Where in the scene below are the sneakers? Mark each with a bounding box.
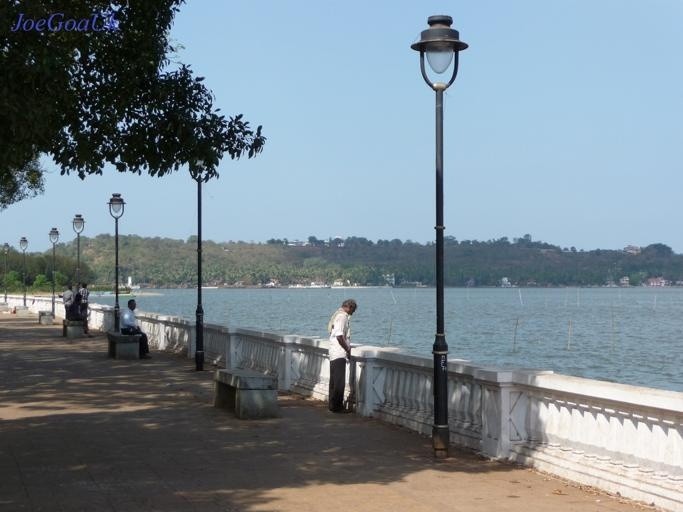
[330,407,351,413]
[140,354,152,359]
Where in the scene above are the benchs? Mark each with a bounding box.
[39,311,52,324]
[215,368,277,418]
[106,331,142,359]
[63,319,85,338]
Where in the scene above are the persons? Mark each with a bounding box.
[327,298,357,414]
[79,284,89,315]
[66,293,88,334]
[62,285,73,312]
[119,299,153,359]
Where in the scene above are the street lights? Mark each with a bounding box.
[106,193,127,333]
[408,13,469,466]
[70,214,87,294]
[3,242,10,303]
[19,236,29,307]
[190,154,214,375]
[47,226,62,318]
